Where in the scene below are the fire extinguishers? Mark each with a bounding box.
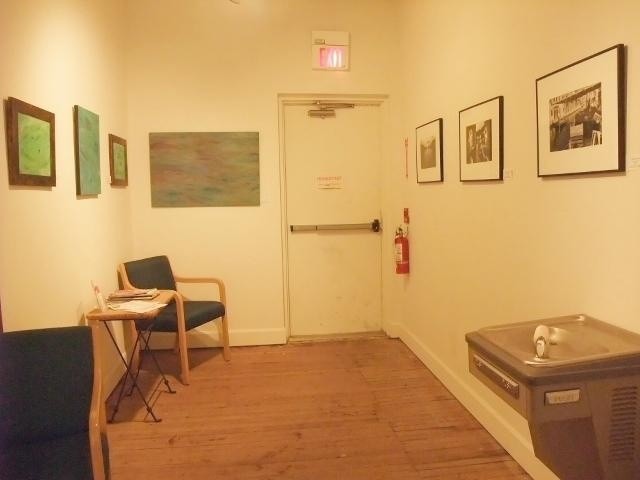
[395,226,409,274]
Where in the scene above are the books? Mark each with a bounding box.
[108,287,168,314]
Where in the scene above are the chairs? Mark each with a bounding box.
[0,326,113,480]
[118,255,230,385]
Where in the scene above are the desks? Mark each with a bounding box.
[87,291,177,422]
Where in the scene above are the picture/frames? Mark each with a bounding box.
[109,134,128,186]
[6,96,56,186]
[459,96,503,182]
[535,43,625,178]
[416,118,442,183]
